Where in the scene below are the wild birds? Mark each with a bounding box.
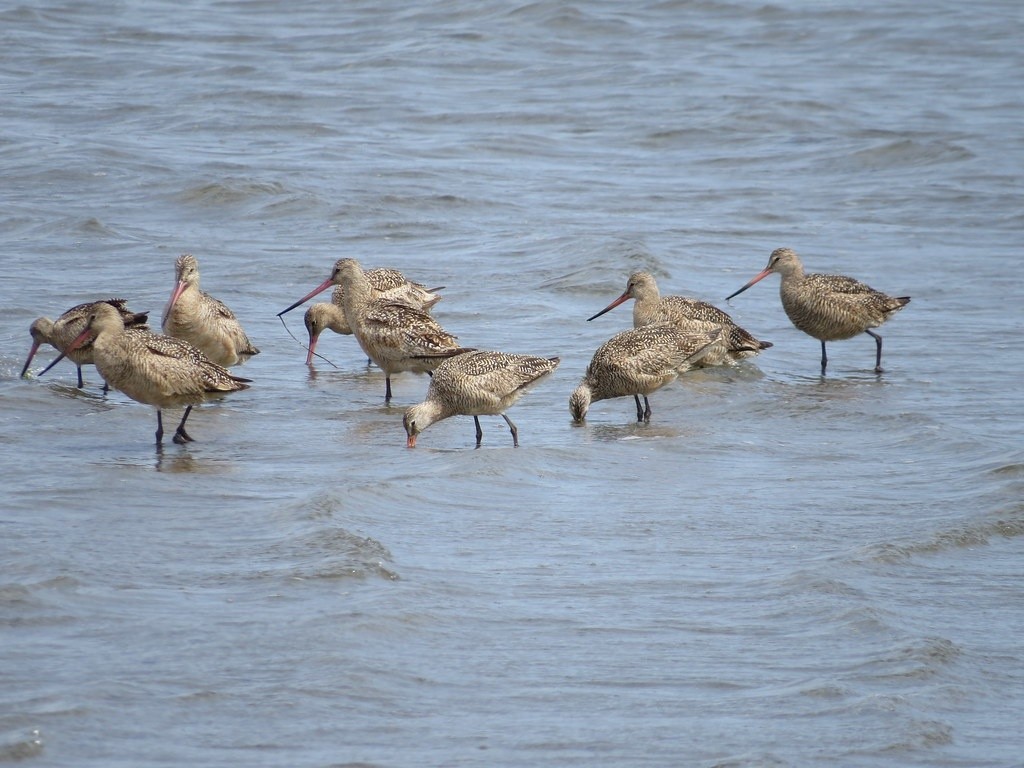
[21,246,910,446]
